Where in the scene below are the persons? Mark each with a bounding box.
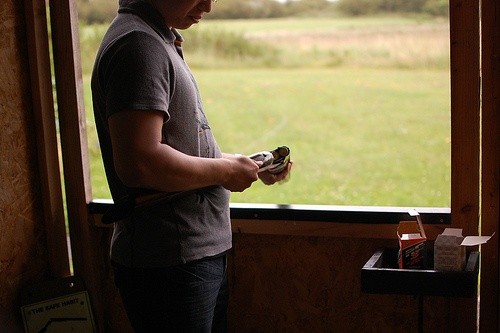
[90,0,292,333]
